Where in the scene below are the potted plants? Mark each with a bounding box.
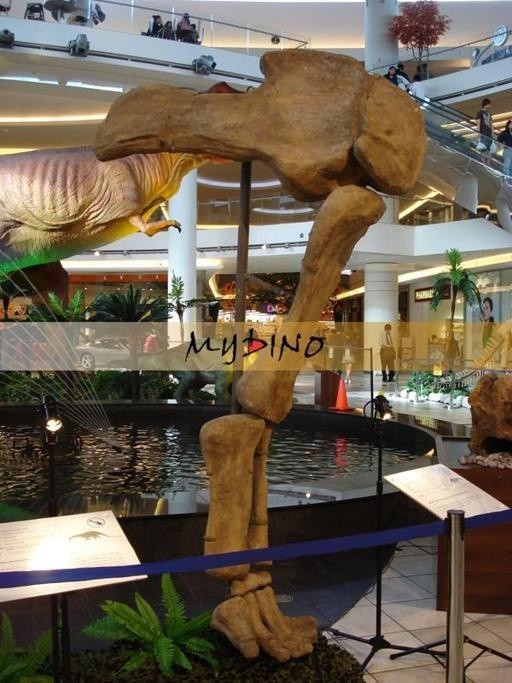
[396,367,473,409]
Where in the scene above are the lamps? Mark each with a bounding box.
[0,27,264,84]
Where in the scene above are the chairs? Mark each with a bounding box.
[138,23,208,45]
[24,2,44,21]
[0,0,12,16]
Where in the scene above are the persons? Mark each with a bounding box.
[143,328,162,353]
[443,332,460,375]
[475,99,494,166]
[476,298,497,349]
[496,120,512,178]
[384,62,432,93]
[315,328,356,386]
[150,12,200,44]
[379,323,398,383]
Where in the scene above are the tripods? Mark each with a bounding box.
[390,635,512,670]
[332,421,447,670]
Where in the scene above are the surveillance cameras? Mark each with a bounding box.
[198,55,216,69]
[75,34,87,54]
[0,29,15,48]
